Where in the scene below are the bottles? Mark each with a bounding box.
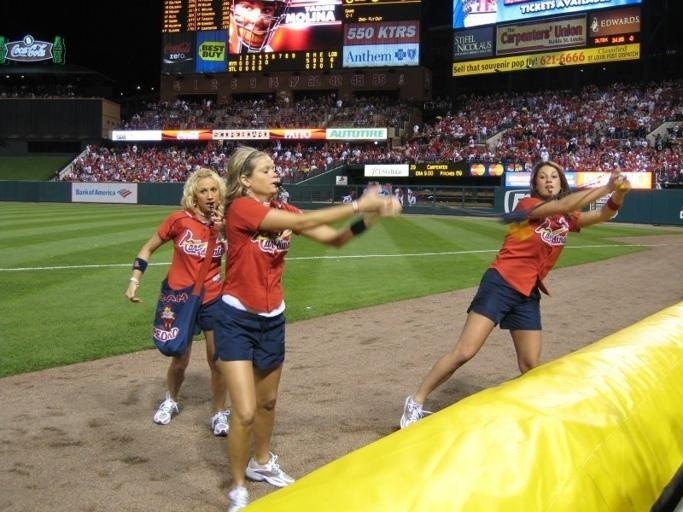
[54,37,63,63]
[0,37,7,66]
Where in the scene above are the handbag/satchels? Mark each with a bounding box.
[152,276,202,358]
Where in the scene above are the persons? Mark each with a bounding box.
[61,83,682,207]
[398,160,633,432]
[212,147,404,512]
[125,166,230,436]
[229,0,290,55]
[2,91,63,99]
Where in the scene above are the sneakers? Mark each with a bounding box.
[212,410,231,436]
[152,390,179,425]
[400,395,434,431]
[245,452,296,488]
[228,488,248,512]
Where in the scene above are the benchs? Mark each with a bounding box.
[63,77,683,213]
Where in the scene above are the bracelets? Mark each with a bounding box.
[606,197,619,211]
[132,258,147,273]
[352,199,360,216]
[129,278,138,285]
[349,217,365,235]
